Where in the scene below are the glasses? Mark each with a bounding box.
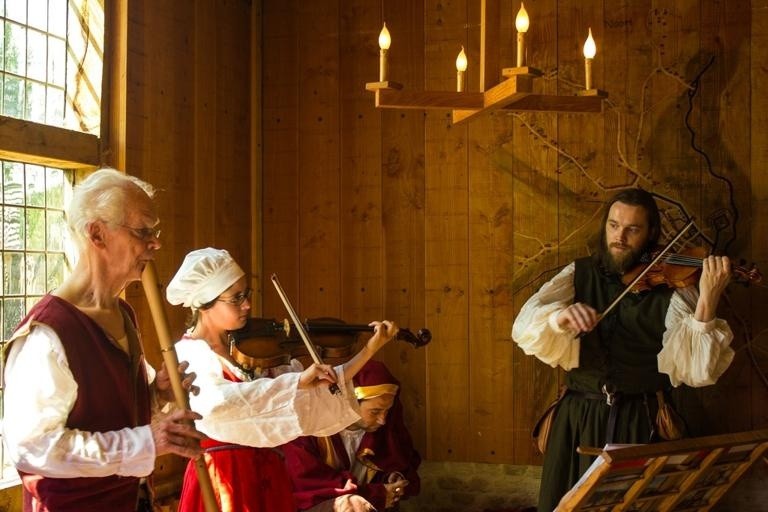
[214,288,253,306]
[101,220,162,242]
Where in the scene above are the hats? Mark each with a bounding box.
[166,247,246,310]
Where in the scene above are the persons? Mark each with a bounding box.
[280,358,423,511]
[509,187,737,512]
[0,166,210,512]
[165,244,401,512]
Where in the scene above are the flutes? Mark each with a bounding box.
[140,260,221,512]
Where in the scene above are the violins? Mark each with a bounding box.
[622,245,764,294]
[226,318,431,371]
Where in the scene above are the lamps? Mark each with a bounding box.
[364,1,609,125]
